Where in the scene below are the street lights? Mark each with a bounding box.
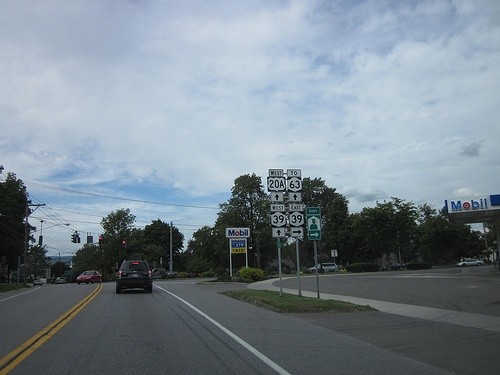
[24,224,70,282]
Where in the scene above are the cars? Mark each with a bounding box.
[382,263,404,271]
[76,271,102,284]
[457,259,484,267]
[32,280,43,286]
[308,262,337,272]
[55,277,66,284]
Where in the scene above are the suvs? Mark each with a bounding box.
[115,259,153,294]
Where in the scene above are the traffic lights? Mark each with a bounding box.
[71,233,81,243]
